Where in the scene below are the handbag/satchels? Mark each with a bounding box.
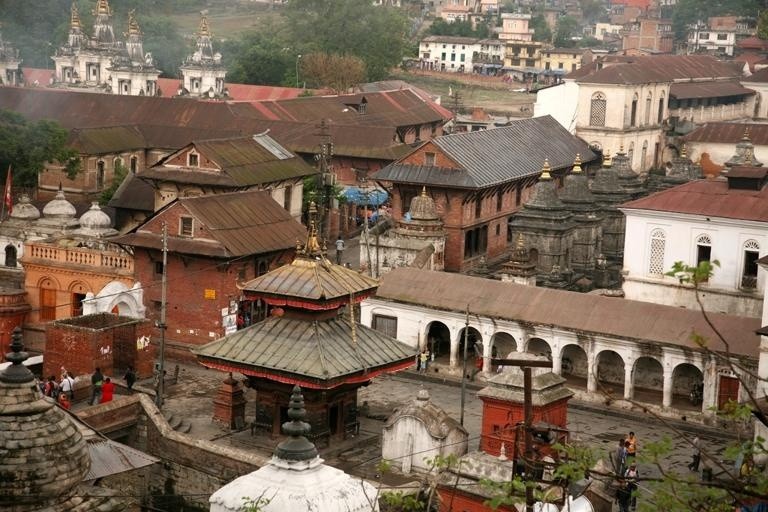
[71,389,74,400]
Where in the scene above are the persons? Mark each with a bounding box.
[334,236,344,265]
[623,461,640,511]
[99,377,116,403]
[420,351,427,370]
[687,432,703,472]
[236,313,245,330]
[153,370,167,407]
[624,431,636,458]
[417,354,421,370]
[123,365,136,395]
[622,441,630,463]
[37,370,75,411]
[616,438,625,471]
[87,367,103,405]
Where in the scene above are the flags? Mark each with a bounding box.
[2,163,13,216]
